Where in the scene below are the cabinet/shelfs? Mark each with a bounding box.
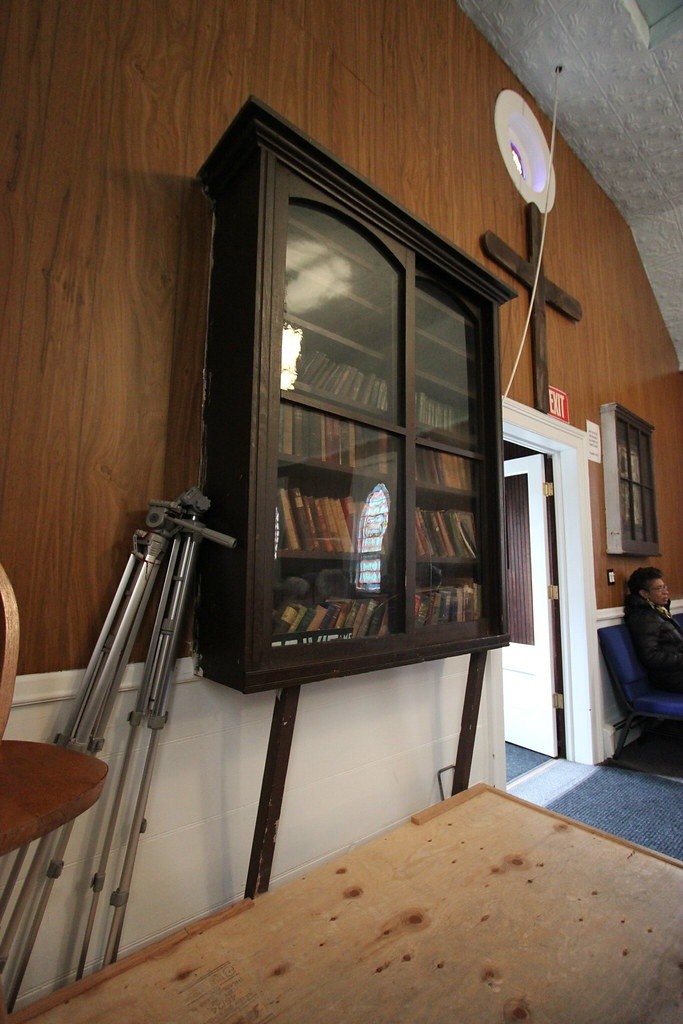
[194,95,519,693]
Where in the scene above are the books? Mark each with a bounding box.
[278,485,478,559]
[279,403,473,491]
[294,345,466,435]
[274,575,482,646]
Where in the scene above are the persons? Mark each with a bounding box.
[622,566,683,696]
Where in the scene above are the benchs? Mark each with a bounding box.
[596,612,683,760]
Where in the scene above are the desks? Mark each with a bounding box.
[1,783,683,1024]
[0,738,112,856]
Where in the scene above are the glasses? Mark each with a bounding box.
[649,585,668,591]
